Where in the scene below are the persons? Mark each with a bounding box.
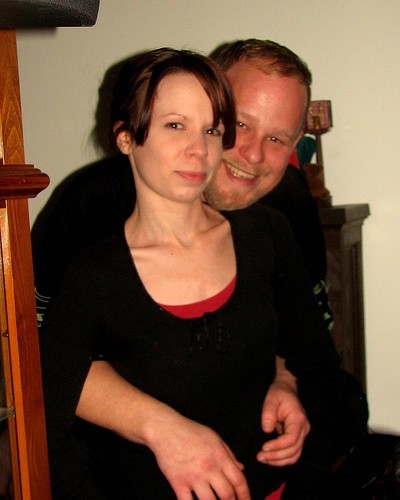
[44,45,329,499]
[1,36,399,500]
